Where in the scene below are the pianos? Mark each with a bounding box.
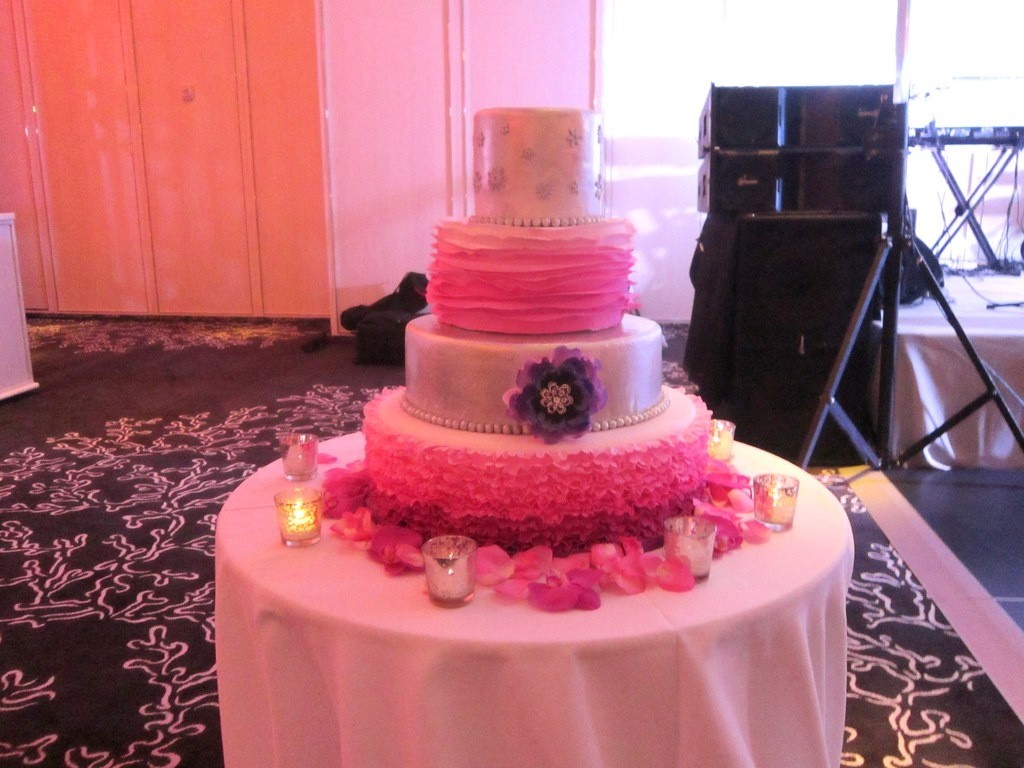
[908,126,1024,149]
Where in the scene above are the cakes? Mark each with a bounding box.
[362,110,714,558]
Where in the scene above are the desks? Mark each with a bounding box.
[214,430,857,768]
